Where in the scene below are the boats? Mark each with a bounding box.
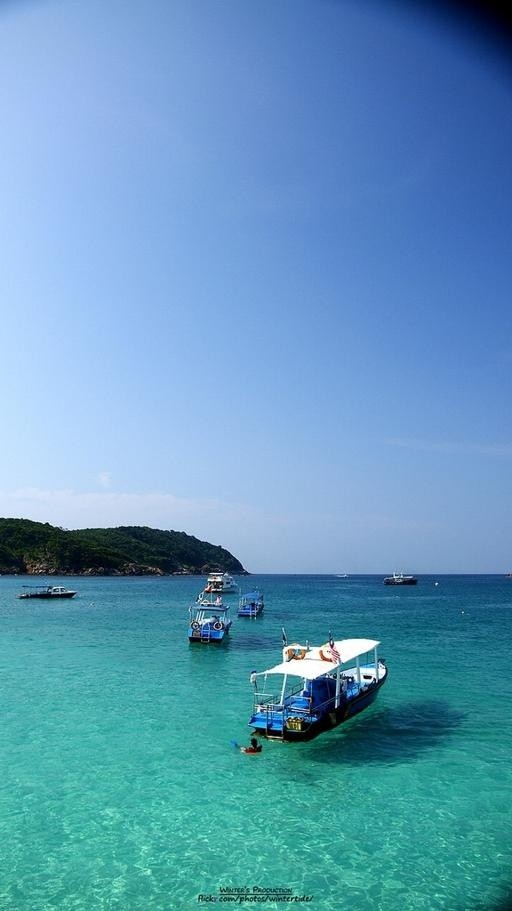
[236,592,264,617]
[383,573,418,586]
[16,586,78,600]
[248,628,388,742]
[207,573,237,592]
[187,586,233,644]
[337,573,349,578]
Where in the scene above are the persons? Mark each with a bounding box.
[332,672,344,686]
[242,738,262,754]
[216,595,222,602]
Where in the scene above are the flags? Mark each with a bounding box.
[329,630,340,661]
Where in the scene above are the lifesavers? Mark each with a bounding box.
[287,644,305,660]
[213,621,223,630]
[191,621,199,630]
[320,644,332,662]
